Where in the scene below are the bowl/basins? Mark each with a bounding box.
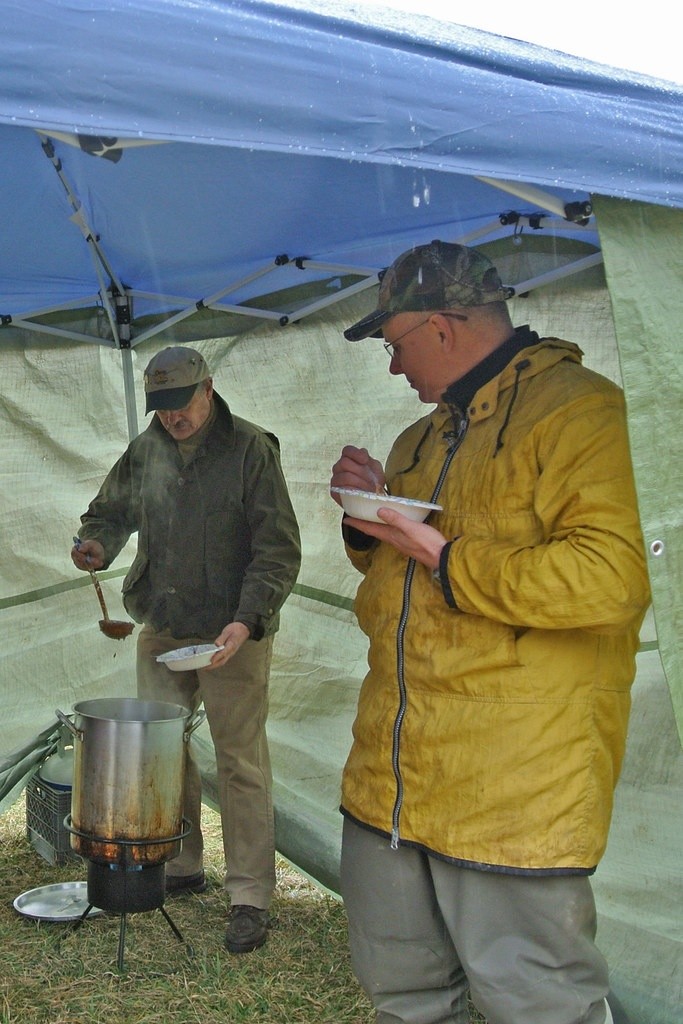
[156,643,225,671]
[331,487,443,524]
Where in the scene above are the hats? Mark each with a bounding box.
[143,344,210,416]
[343,241,516,342]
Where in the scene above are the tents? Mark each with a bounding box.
[0,0,683,1024]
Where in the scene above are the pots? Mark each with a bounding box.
[44,699,206,864]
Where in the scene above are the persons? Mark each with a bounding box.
[71,348,301,953]
[330,243,652,1024]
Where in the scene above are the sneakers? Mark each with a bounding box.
[225,903,269,953]
[162,870,208,895]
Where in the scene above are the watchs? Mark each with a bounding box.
[432,569,442,587]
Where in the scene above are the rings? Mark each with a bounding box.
[74,560,76,564]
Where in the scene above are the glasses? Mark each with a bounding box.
[383,313,468,358]
